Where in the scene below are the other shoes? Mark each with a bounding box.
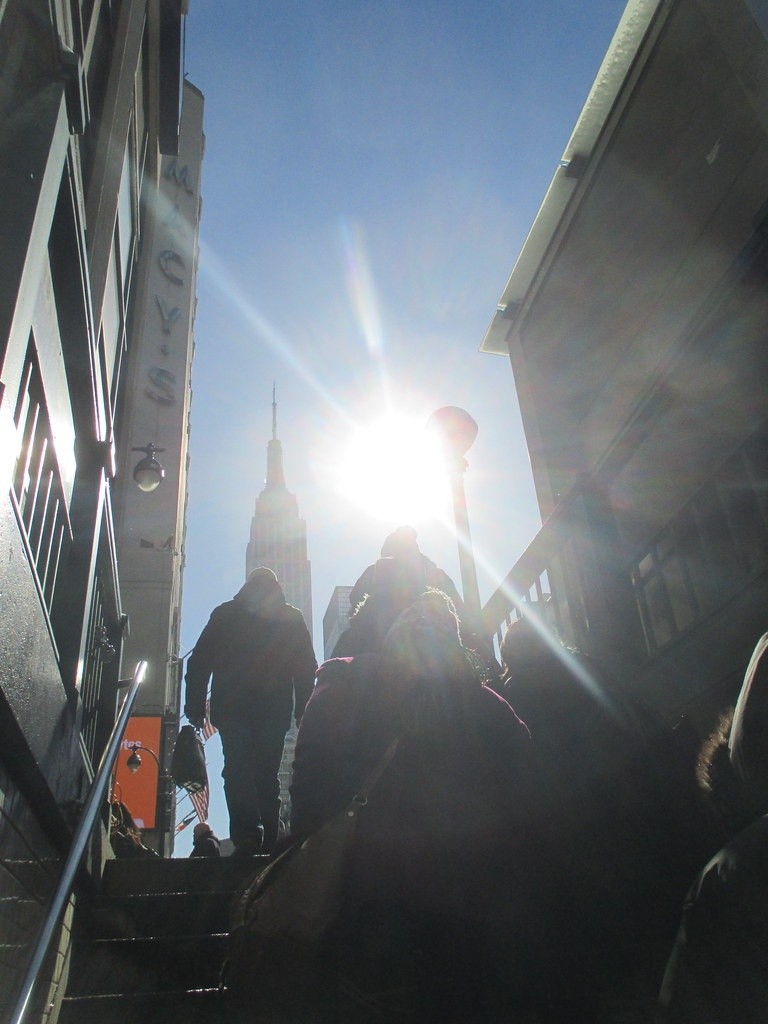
[229,831,263,857]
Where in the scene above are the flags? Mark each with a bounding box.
[176,816,196,831]
[202,697,219,742]
[189,780,209,823]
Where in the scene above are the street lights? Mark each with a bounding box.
[125,743,169,859]
[430,407,481,615]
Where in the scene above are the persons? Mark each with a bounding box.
[289,523,768,1024]
[184,567,318,855]
[189,823,220,858]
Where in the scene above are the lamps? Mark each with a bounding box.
[130,444,167,493]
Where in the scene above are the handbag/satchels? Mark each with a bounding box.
[224,792,369,942]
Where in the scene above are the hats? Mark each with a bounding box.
[193,823,212,837]
[380,525,420,557]
[499,617,545,661]
[247,566,277,582]
[383,585,462,648]
